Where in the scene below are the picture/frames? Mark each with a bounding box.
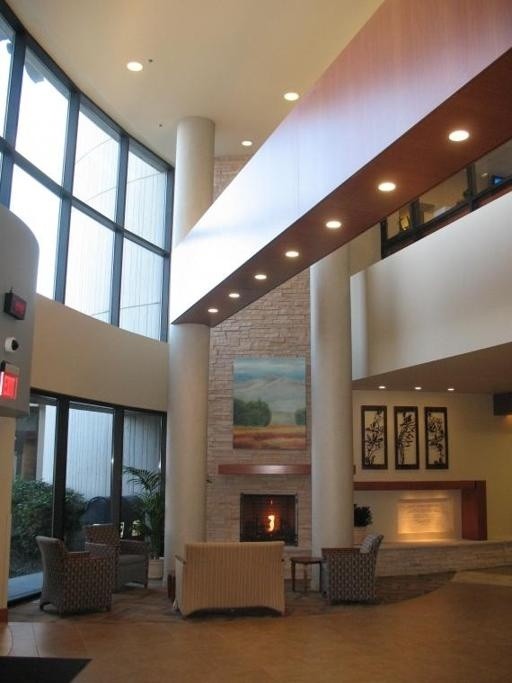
[360,404,448,470]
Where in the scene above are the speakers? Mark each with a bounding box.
[493,390,512,415]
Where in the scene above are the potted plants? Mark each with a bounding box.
[122,464,165,580]
[353,503,373,545]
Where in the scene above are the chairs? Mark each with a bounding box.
[321,533,384,606]
[35,522,150,618]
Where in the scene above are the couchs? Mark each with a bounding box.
[172,541,286,619]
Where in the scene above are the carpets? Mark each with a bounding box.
[0,655,92,682]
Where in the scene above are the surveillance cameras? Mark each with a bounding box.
[5,337,20,352]
